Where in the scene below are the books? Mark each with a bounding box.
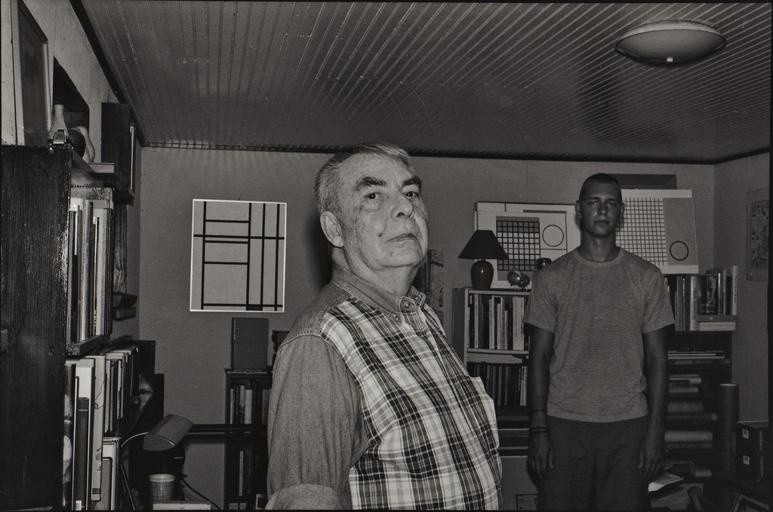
[660,265,740,479]
[230,384,272,511]
[465,293,528,407]
[230,318,268,371]
[63,197,138,510]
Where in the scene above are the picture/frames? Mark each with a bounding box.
[188,197,288,314]
[9,0,53,147]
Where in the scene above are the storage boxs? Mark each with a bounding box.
[735,418,771,486]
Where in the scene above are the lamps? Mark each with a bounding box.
[119,413,196,511]
[611,19,727,67]
[453,230,511,291]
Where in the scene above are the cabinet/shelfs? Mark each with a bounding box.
[663,321,735,446]
[2,143,167,509]
[453,285,554,426]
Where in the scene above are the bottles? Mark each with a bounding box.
[47,104,68,144]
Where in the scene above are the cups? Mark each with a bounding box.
[147,472,174,506]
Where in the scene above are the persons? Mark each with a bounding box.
[267,143,503,509]
[523,174,675,511]
